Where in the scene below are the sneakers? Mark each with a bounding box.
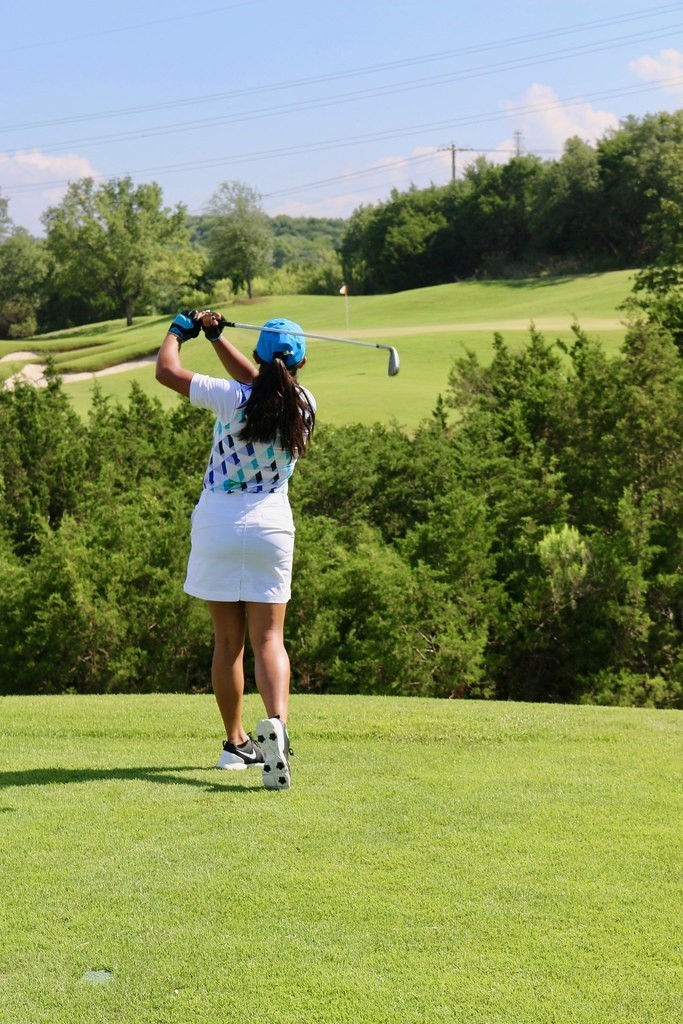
[216,731,265,770]
[257,714,294,790]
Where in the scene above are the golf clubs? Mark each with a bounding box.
[220,318,401,379]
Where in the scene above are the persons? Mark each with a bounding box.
[155,310,317,795]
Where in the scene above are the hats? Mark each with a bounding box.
[256,318,307,367]
